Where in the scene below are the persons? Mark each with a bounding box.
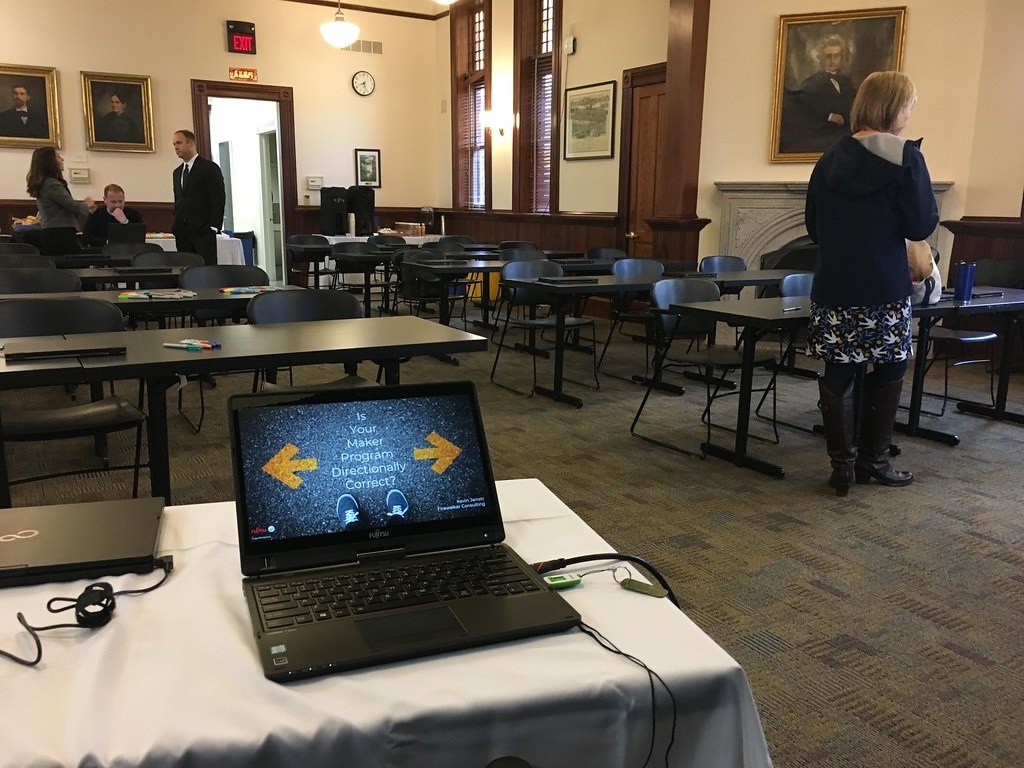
[26,146,98,232]
[82,184,143,247]
[805,71,940,497]
[172,130,226,266]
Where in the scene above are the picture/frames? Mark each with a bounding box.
[355,148,381,188]
[0,63,62,150]
[561,80,617,160]
[769,6,909,163]
[80,70,155,155]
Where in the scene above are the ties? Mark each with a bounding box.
[183,164,189,188]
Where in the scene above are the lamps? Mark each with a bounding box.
[320,0,360,51]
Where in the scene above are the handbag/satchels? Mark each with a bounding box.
[905,238,941,307]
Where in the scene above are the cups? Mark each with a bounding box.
[954,260,977,302]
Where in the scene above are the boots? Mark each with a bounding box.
[818,377,857,497]
[855,371,914,485]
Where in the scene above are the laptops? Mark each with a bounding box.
[367,243,468,266]
[548,259,594,264]
[114,266,172,273]
[4,336,126,360]
[0,497,167,588]
[942,288,1004,298]
[662,271,717,278]
[108,223,147,245]
[228,381,582,683]
[539,277,599,284]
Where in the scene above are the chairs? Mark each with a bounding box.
[755,273,836,431]
[0,225,749,453]
[894,324,997,417]
[630,277,780,460]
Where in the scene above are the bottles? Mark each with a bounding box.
[395,222,425,236]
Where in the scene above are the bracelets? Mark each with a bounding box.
[124,219,129,224]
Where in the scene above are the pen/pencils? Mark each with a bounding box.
[163,339,222,352]
[783,306,802,312]
[117,289,197,299]
[219,286,284,293]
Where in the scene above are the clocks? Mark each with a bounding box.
[351,71,376,96]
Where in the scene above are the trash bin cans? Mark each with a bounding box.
[222,229,255,266]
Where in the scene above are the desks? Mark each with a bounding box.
[399,261,617,366]
[331,251,583,315]
[0,313,488,506]
[1,478,773,768]
[503,269,804,407]
[319,234,447,264]
[0,284,312,333]
[146,236,246,265]
[63,266,191,288]
[288,244,501,291]
[668,288,1024,478]
